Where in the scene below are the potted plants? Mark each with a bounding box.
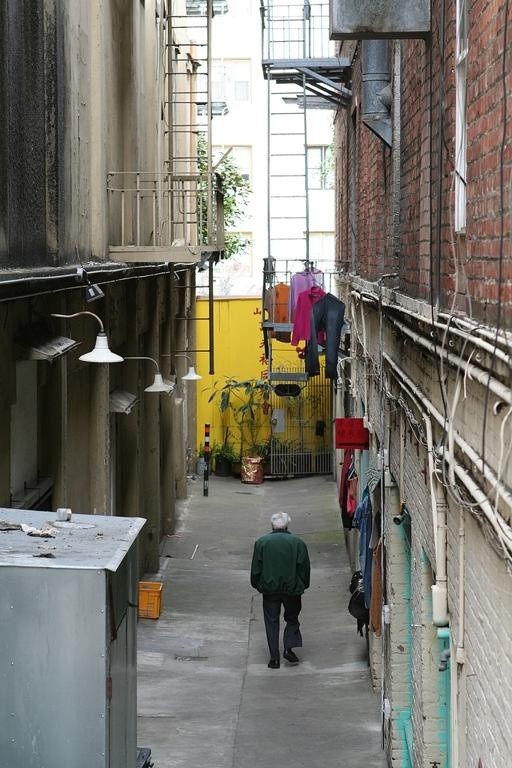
[196,376,331,483]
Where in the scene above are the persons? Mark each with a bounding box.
[249,512,312,669]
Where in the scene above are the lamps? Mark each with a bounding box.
[393,502,408,525]
[123,355,175,393]
[51,311,122,365]
[174,351,202,381]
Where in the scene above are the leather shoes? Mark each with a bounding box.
[267,660,281,668]
[284,649,299,664]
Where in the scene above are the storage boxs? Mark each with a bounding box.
[138,581,163,620]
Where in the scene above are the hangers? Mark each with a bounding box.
[348,455,357,469]
[307,278,324,298]
[302,261,313,273]
[349,467,360,479]
[276,275,287,284]
